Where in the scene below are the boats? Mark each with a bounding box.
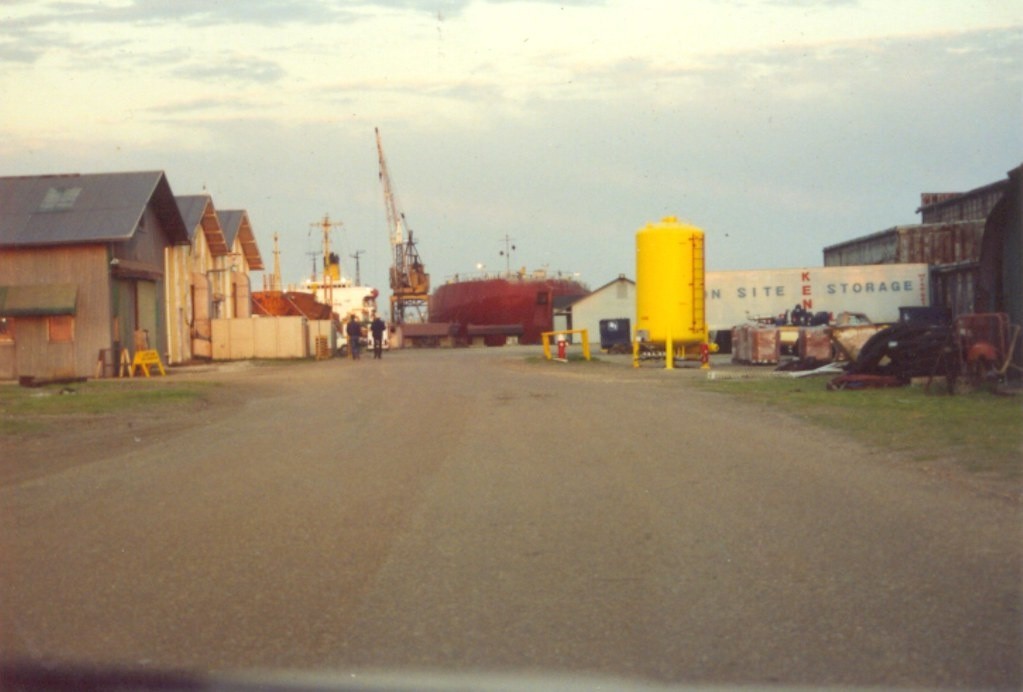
[429,232,595,347]
[283,212,379,323]
[251,232,343,333]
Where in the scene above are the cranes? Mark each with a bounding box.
[373,126,430,295]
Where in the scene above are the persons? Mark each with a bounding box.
[346,313,361,360]
[371,313,386,358]
